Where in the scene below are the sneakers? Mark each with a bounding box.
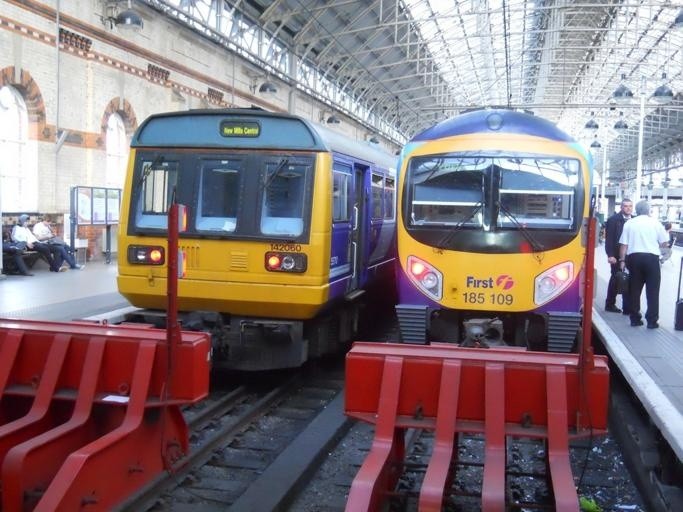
[59,266,66,272]
[623,310,630,315]
[631,321,643,326]
[648,323,659,328]
[23,271,33,275]
[605,304,621,312]
[10,245,22,255]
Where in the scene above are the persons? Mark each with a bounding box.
[656,223,672,266]
[619,199,670,328]
[605,199,636,315]
[11,214,69,272]
[3,231,35,276]
[33,214,85,272]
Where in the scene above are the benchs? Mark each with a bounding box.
[2,223,77,272]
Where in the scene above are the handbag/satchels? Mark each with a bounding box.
[614,272,629,294]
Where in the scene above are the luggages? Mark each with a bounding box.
[674,256,683,330]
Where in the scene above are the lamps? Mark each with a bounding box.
[101,8,142,30]
[584,70,673,147]
[364,132,379,144]
[253,78,277,94]
[322,111,341,123]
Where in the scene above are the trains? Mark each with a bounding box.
[394,108,599,354]
[118,104,397,372]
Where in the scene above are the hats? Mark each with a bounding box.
[19,214,28,223]
